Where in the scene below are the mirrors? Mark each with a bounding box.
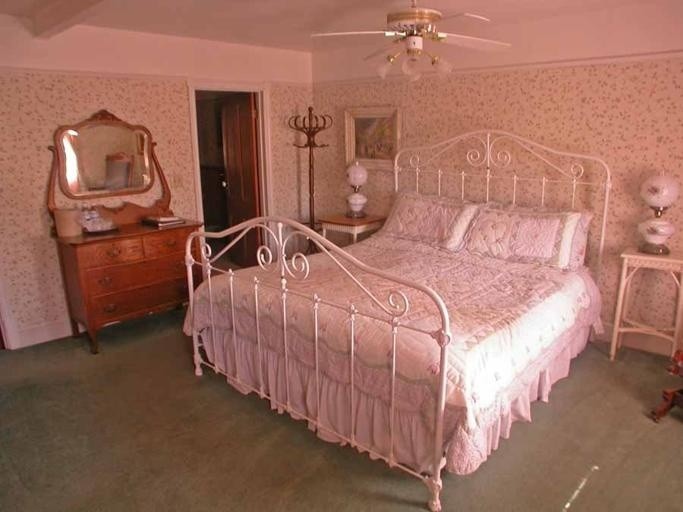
[53,109,156,199]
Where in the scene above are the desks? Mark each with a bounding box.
[607,248,683,360]
[318,214,383,243]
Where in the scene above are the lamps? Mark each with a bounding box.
[373,35,450,82]
[346,163,368,218]
[640,171,680,259]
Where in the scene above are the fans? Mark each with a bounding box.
[309,0,511,82]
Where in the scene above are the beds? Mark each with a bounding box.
[180,129,612,512]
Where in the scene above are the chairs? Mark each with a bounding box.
[103,149,133,192]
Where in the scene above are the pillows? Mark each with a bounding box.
[388,192,479,251]
[461,202,589,269]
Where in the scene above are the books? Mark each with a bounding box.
[144,216,181,221]
[141,220,184,227]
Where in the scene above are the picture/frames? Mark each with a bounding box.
[345,107,401,172]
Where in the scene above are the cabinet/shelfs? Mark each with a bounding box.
[55,218,203,355]
